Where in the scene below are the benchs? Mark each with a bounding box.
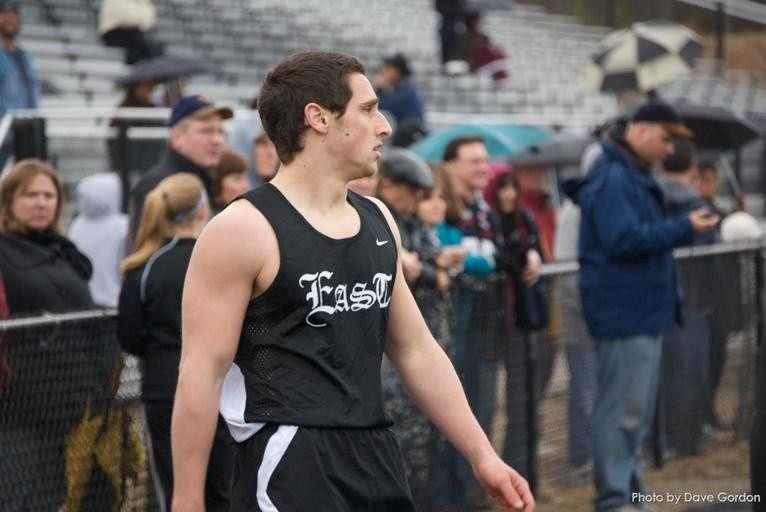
[1,1,765,234]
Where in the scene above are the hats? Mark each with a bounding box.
[378,147,436,192]
[629,102,695,138]
[381,54,412,77]
[166,93,234,128]
